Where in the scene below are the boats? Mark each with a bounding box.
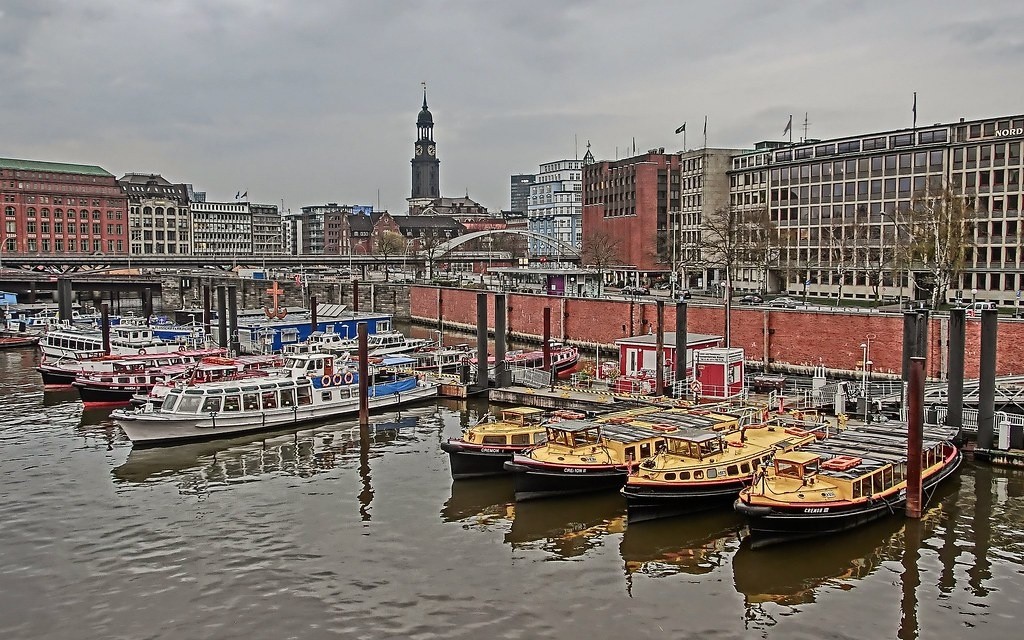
[441,401,628,479]
[733,422,965,537]
[0,296,582,454]
[504,405,742,501]
[620,409,827,526]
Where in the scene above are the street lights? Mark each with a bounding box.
[487,226,493,268]
[347,237,352,281]
[668,210,682,302]
[403,237,420,283]
[880,211,902,313]
[553,219,564,268]
[262,234,281,273]
[570,277,574,296]
[971,288,978,316]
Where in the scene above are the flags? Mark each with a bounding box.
[912,94,916,122]
[241,190,247,198]
[676,124,685,134]
[235,192,239,199]
[783,120,790,136]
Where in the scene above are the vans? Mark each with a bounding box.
[965,303,997,317]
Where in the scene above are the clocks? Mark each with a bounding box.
[415,145,423,156]
[427,145,436,157]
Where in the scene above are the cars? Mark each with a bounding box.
[739,295,764,306]
[653,283,661,290]
[661,283,679,290]
[670,290,691,300]
[769,297,797,307]
[633,288,650,295]
[620,286,636,294]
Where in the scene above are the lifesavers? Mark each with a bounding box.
[178,345,186,352]
[321,375,333,387]
[690,380,702,393]
[138,349,147,355]
[187,345,194,350]
[344,372,354,384]
[333,374,344,386]
